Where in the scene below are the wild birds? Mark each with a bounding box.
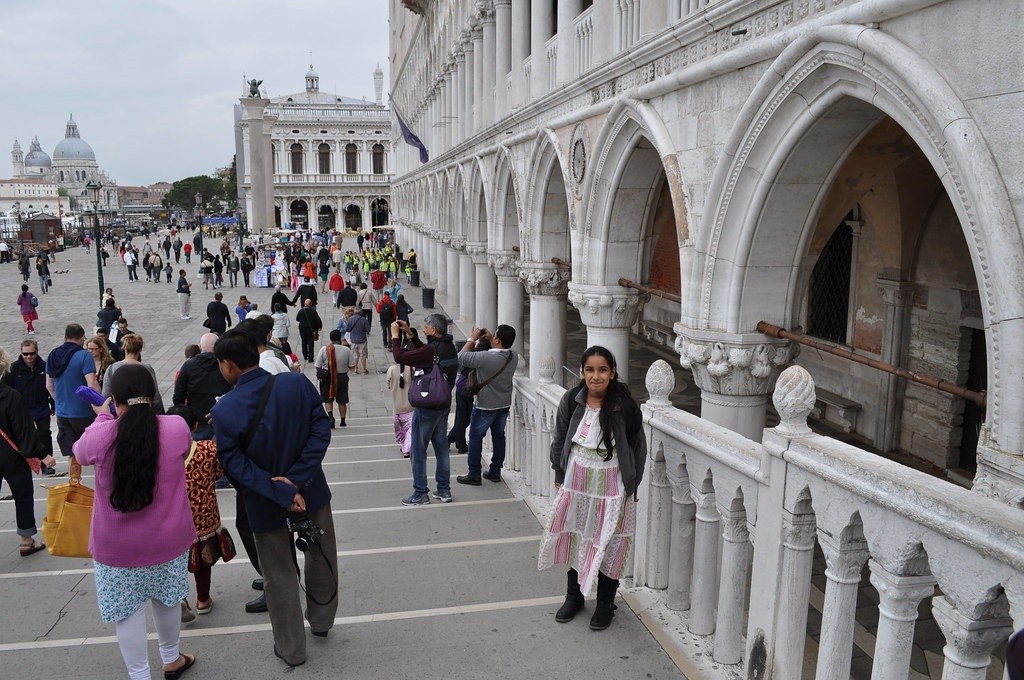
[54,269,71,275]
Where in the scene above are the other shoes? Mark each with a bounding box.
[330,417,335,428]
[181,314,192,319]
[458,445,468,454]
[42,466,55,475]
[333,303,336,307]
[129,276,160,283]
[26,331,35,334]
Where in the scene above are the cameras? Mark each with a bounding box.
[286,509,324,551]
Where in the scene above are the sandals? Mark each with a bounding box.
[19,539,45,556]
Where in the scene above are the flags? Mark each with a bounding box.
[392,104,429,164]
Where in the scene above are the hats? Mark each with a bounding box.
[240,295,246,300]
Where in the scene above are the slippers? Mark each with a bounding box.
[164,578,328,680]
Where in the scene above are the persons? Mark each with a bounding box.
[84,235,92,252]
[0,314,356,680]
[538,345,647,630]
[17,284,39,334]
[95,220,417,374]
[386,314,518,506]
[0,232,64,293]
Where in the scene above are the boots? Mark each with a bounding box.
[556,569,584,623]
[590,570,620,630]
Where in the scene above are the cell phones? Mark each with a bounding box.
[478,329,486,336]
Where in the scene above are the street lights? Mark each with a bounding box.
[14,201,24,252]
[86,179,104,309]
[236,206,244,252]
[194,191,203,263]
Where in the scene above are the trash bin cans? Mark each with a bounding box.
[410,271,420,286]
[422,288,435,308]
[401,260,408,272]
[398,253,403,263]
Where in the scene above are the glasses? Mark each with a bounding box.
[22,351,36,356]
[83,336,86,340]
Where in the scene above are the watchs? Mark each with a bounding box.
[466,338,476,344]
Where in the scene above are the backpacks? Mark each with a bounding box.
[380,299,393,322]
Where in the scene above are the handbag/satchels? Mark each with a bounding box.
[40,456,94,558]
[132,259,154,269]
[25,457,42,475]
[408,343,477,409]
[313,331,319,341]
[48,278,52,286]
[203,318,211,329]
[316,368,330,383]
[31,296,38,307]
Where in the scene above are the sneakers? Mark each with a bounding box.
[401,490,452,506]
[457,469,501,485]
